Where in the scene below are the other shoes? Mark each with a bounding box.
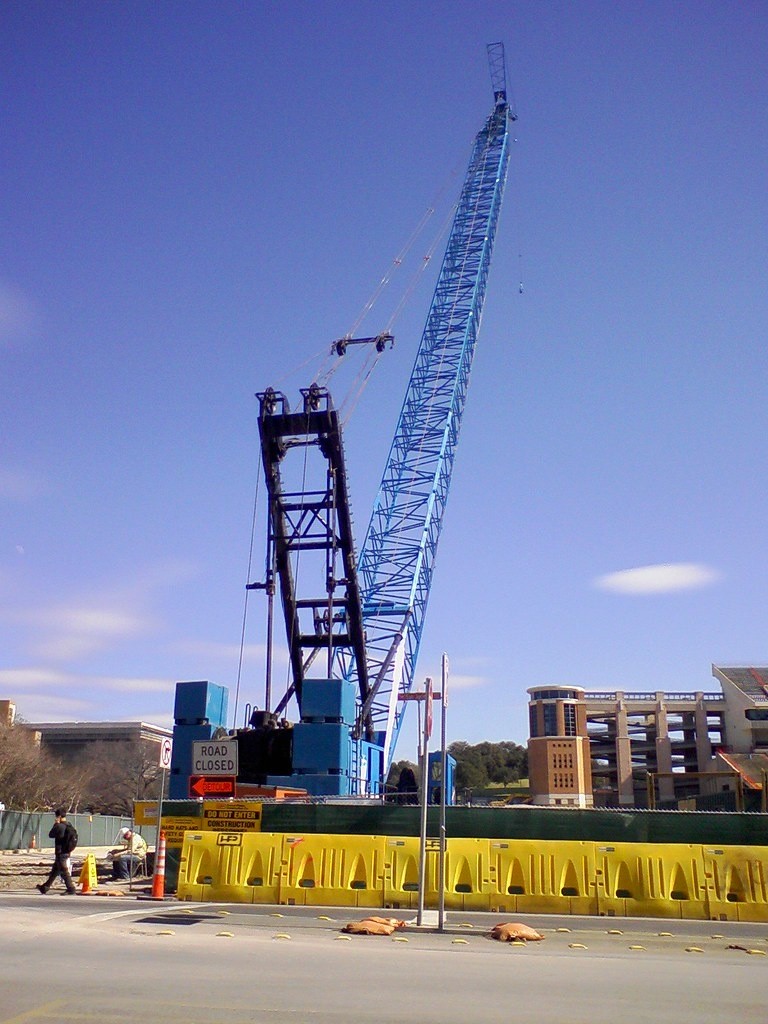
[116,876,130,882]
[60,888,78,896]
[36,883,48,895]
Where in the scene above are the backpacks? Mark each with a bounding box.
[58,821,78,854]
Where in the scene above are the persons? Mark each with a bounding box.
[106,826,147,882]
[36,807,78,896]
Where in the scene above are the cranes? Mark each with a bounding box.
[186,38,518,800]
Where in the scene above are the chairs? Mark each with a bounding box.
[130,857,147,876]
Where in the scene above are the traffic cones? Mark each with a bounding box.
[29,833,36,849]
[137,829,177,900]
[76,855,101,895]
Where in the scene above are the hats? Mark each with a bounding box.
[119,827,130,838]
